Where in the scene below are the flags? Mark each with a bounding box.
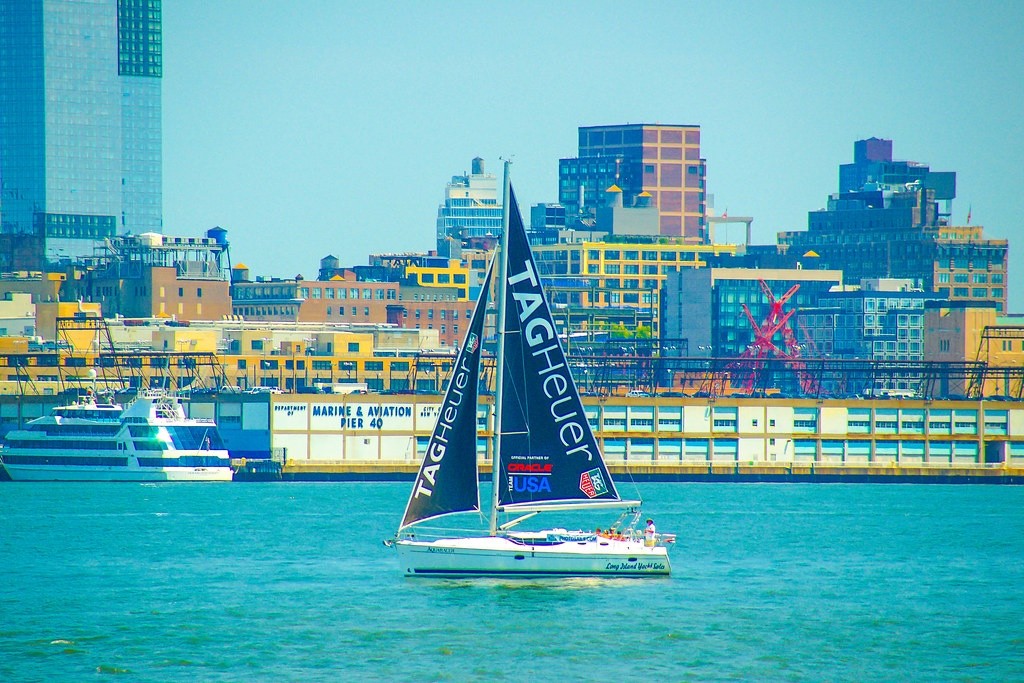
[722,210,727,218]
[967,205,972,224]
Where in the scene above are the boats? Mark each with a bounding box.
[0,388,234,482]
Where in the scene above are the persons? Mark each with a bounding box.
[596,528,625,541]
[644,518,655,547]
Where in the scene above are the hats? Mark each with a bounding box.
[646,518,653,523]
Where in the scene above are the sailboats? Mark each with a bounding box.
[383,157,674,580]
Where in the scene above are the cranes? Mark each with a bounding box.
[735,276,827,394]
